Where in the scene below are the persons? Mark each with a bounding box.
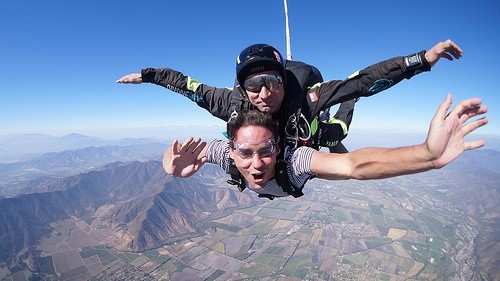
[163,93,490,201]
[114,38,463,152]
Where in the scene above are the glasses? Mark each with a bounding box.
[243,73,283,93]
[232,139,277,159]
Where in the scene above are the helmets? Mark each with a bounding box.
[236,42,286,85]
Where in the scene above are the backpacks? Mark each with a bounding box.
[227,61,322,138]
